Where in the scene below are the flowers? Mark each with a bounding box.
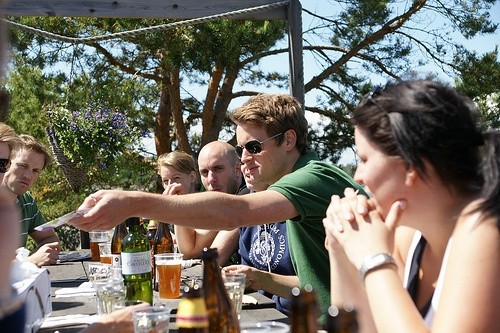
[44,101,151,171]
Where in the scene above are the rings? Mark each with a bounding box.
[90,194,97,202]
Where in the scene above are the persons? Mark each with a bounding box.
[67,93,371,333]
[157,151,205,195]
[209,162,297,315]
[162,142,250,268]
[323,78,500,333]
[0,123,62,296]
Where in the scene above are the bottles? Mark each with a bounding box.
[112,222,129,269]
[175,285,209,333]
[151,221,173,292]
[200,248,241,333]
[146,219,159,288]
[121,217,153,308]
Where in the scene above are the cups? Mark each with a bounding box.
[238,321,291,333]
[109,265,122,281]
[131,305,172,333]
[98,242,112,265]
[221,272,246,318]
[88,230,110,263]
[89,264,112,288]
[93,279,126,316]
[153,253,184,300]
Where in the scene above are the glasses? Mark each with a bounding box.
[0,158,11,172]
[235,131,285,159]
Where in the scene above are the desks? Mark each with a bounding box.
[36,248,289,333]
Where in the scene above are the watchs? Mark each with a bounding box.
[360,254,398,281]
